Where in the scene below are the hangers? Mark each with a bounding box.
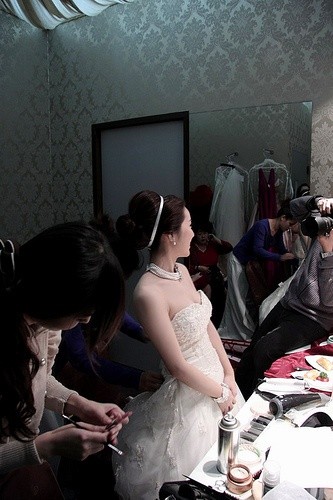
[216,154,249,175]
[249,150,286,172]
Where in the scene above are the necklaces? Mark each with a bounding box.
[146,263,181,280]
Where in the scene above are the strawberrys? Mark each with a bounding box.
[317,372,329,382]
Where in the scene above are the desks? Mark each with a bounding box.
[186,341,333,500]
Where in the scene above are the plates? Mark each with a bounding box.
[291,370,333,392]
[305,355,333,372]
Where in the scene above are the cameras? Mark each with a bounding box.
[300,204,333,237]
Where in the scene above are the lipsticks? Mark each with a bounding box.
[104,413,128,431]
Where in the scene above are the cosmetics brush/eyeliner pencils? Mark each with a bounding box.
[61,414,126,457]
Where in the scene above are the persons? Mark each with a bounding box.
[217,205,302,342]
[234,194,321,402]
[183,222,233,329]
[289,183,312,275]
[0,219,155,500]
[111,189,246,500]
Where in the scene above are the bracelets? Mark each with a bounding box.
[213,383,231,404]
[196,265,199,272]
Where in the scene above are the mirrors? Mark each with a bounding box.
[188,100,313,248]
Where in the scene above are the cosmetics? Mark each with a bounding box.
[224,463,254,495]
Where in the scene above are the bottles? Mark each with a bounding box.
[262,466,281,498]
[217,412,240,474]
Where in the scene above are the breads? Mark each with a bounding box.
[304,370,319,379]
[316,357,333,371]
